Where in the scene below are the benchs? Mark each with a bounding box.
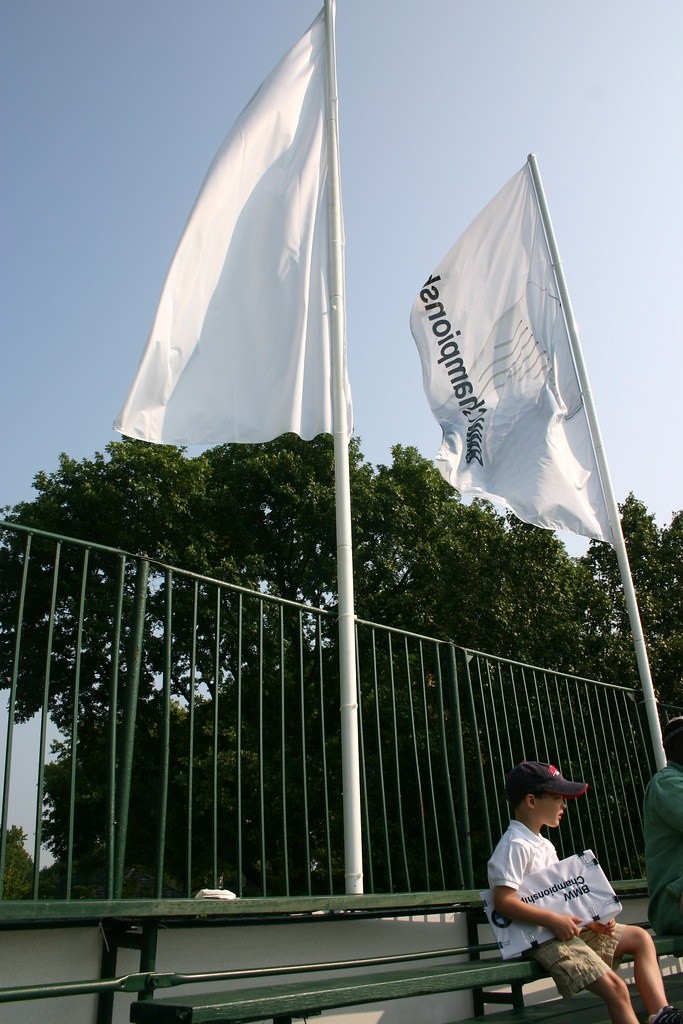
[0,878,683,1024]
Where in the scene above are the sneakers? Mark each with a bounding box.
[648,1005,683,1024]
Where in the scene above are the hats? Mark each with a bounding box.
[507,760,589,803]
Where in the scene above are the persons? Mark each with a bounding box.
[488,762,683,1024]
[643,716,683,934]
